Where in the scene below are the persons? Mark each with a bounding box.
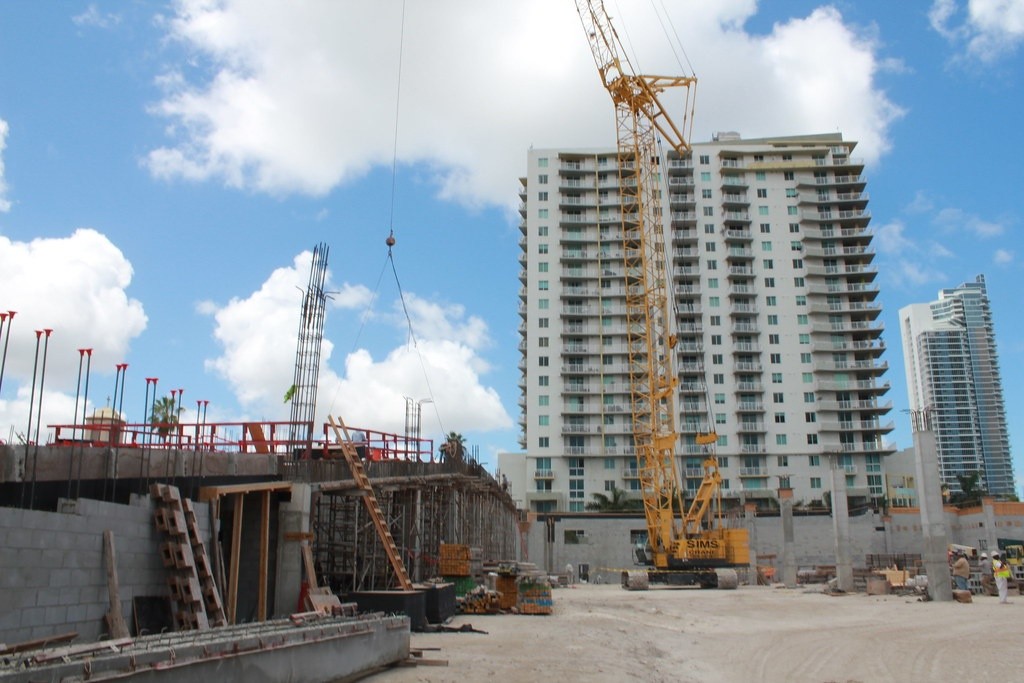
[949,552,971,590]
[978,553,994,595]
[989,550,1011,604]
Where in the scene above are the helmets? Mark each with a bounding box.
[980,553,989,558]
[991,551,1000,557]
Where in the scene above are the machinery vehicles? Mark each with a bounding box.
[571,0,750,590]
